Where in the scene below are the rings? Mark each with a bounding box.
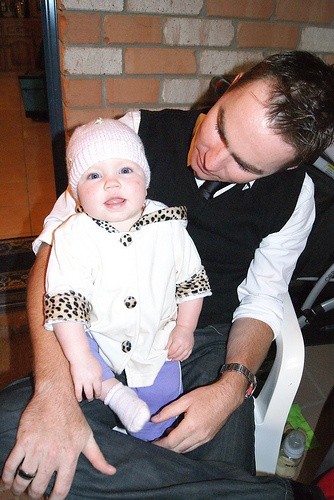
[16,466,36,481]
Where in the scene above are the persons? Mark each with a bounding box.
[0,50,334,500]
[42,116,212,443]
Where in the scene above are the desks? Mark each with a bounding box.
[18,72,49,121]
[0,17,43,69]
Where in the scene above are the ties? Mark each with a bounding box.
[198,180,233,201]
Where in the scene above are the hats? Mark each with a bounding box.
[65,109,150,205]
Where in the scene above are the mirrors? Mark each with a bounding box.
[0,1,71,263]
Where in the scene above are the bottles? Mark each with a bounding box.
[275,430,306,479]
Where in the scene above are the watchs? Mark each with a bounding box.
[218,363,258,401]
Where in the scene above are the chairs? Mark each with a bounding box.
[253,294,306,477]
